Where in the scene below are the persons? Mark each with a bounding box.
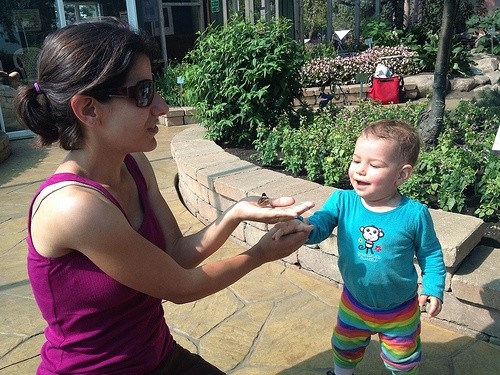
[14,16,319,375]
[271,118,448,375]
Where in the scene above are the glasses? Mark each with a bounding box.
[105,71,157,108]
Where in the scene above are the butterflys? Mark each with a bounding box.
[258,192,269,206]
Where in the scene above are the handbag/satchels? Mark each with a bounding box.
[368,76,405,105]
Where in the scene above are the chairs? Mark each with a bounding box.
[13,46,43,85]
